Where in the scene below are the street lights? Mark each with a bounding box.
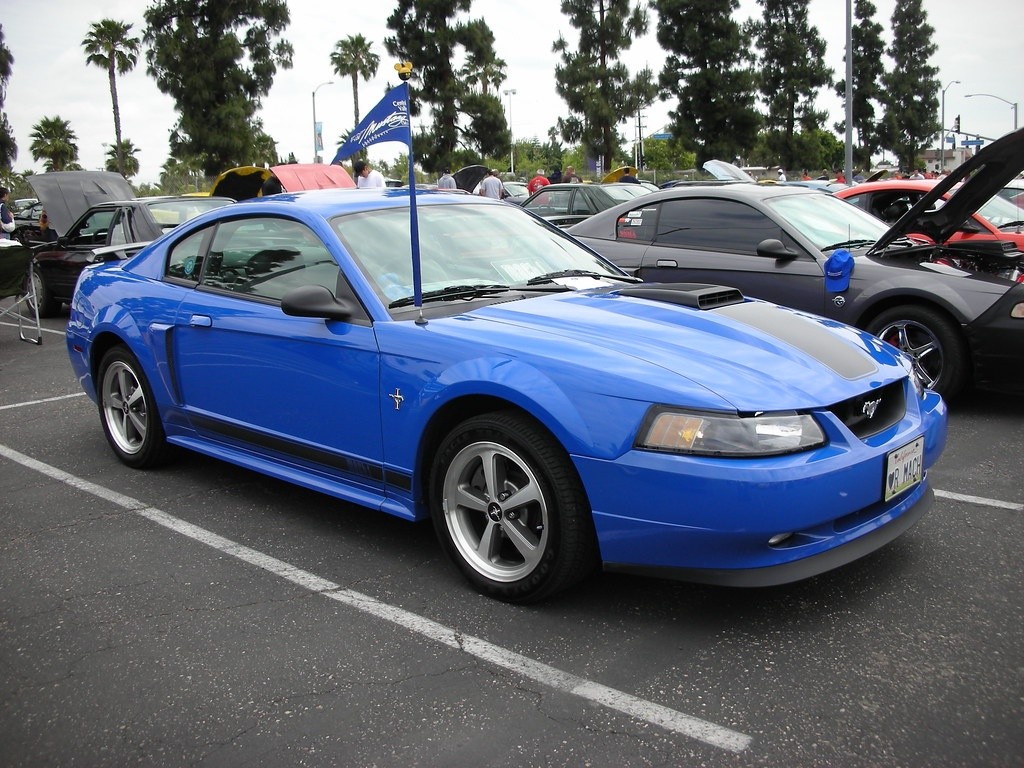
[310,79,335,160]
[964,92,1020,129]
[940,79,961,174]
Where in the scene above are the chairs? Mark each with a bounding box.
[886,201,909,226]
[0,246,42,345]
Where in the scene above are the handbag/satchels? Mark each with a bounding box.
[0,203,15,233]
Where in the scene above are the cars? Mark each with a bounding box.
[834,180,1024,253]
[568,128,1024,407]
[20,172,239,318]
[64,186,949,605]
[521,181,655,229]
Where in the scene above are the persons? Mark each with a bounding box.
[262,161,298,195]
[835,168,845,183]
[438,166,458,189]
[0,187,15,239]
[479,168,506,200]
[562,166,584,183]
[909,163,952,181]
[895,171,902,179]
[800,171,813,180]
[778,168,787,181]
[549,169,564,184]
[619,168,642,184]
[816,169,829,180]
[353,161,387,187]
[527,168,554,206]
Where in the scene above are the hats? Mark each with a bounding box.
[823,248,855,292]
[486,169,493,175]
[778,169,783,173]
[443,168,450,174]
[353,161,365,178]
